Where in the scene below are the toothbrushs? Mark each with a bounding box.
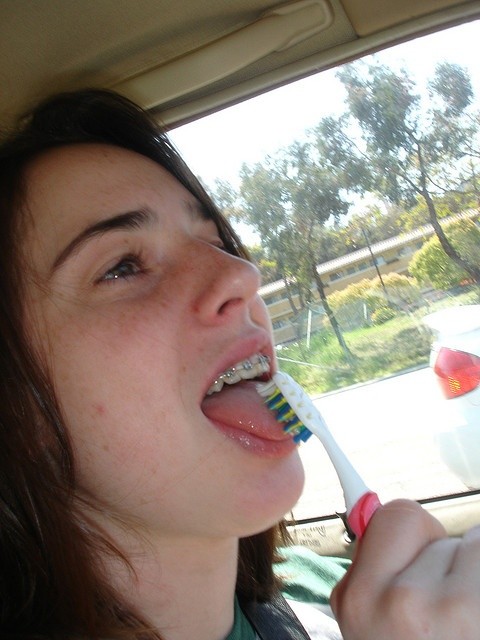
[257,370,382,540]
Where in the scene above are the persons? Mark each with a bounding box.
[0,88,480,638]
